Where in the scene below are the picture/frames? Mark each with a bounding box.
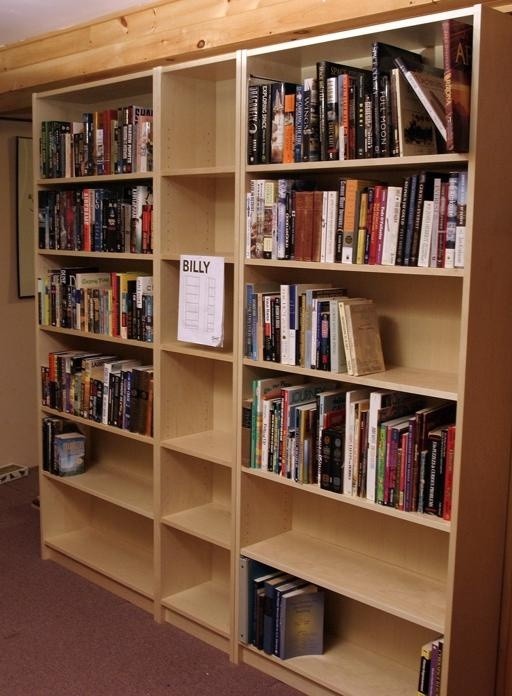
[16,136,34,299]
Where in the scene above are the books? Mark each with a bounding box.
[416,638,444,696]
[42,417,87,477]
[38,267,154,343]
[246,170,467,269]
[236,557,324,660]
[248,19,474,165]
[246,282,386,376]
[38,108,153,179]
[242,376,456,521]
[435,639,443,696]
[41,349,154,438]
[38,183,154,254]
[428,638,442,696]
